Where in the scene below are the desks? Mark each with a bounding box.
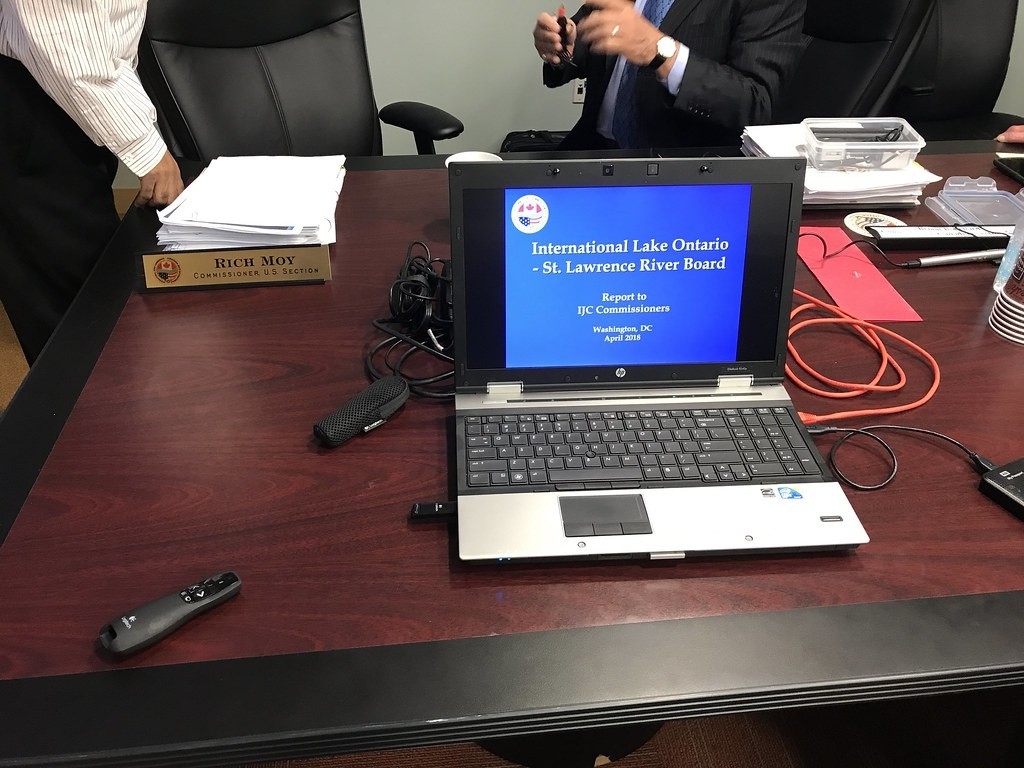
[0,140,1024,768]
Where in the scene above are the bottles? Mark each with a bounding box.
[993,213,1024,293]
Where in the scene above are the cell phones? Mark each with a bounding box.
[994,157,1024,187]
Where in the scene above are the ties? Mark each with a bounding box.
[612,0,676,148]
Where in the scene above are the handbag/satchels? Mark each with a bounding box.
[499,130,572,151]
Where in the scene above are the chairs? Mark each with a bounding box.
[136,0,464,163]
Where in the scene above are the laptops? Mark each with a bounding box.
[447,154,870,570]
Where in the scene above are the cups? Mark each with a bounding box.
[988,242,1024,345]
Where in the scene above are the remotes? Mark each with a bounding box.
[99,570,242,656]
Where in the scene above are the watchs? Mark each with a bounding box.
[648,35,679,72]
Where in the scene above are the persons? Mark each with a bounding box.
[993,125,1024,144]
[533,0,810,161]
[0,0,185,371]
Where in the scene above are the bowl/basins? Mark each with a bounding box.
[802,118,926,171]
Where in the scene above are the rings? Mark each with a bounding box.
[612,24,620,35]
